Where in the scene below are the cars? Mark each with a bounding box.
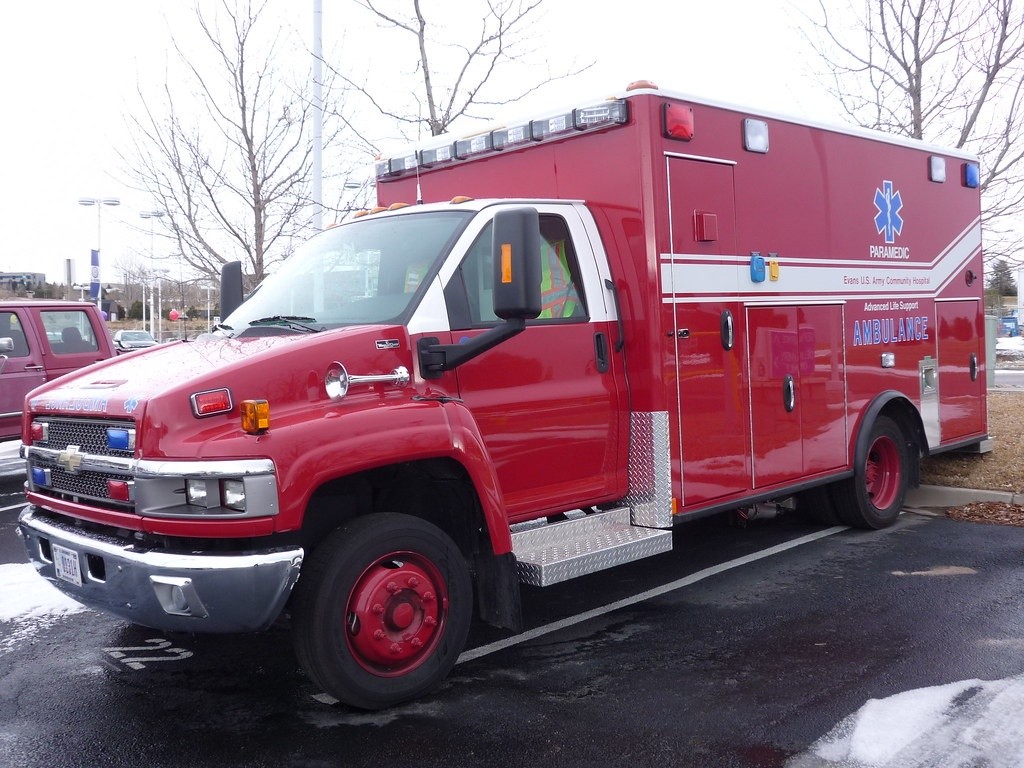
[0,296,119,442]
[111,329,160,358]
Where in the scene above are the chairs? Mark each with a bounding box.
[51,326,93,353]
[0,330,28,356]
[538,240,584,320]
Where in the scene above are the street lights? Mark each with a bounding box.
[77,198,121,311]
[151,266,170,342]
[74,284,92,336]
[139,210,166,338]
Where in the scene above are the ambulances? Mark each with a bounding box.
[16,82,992,712]
